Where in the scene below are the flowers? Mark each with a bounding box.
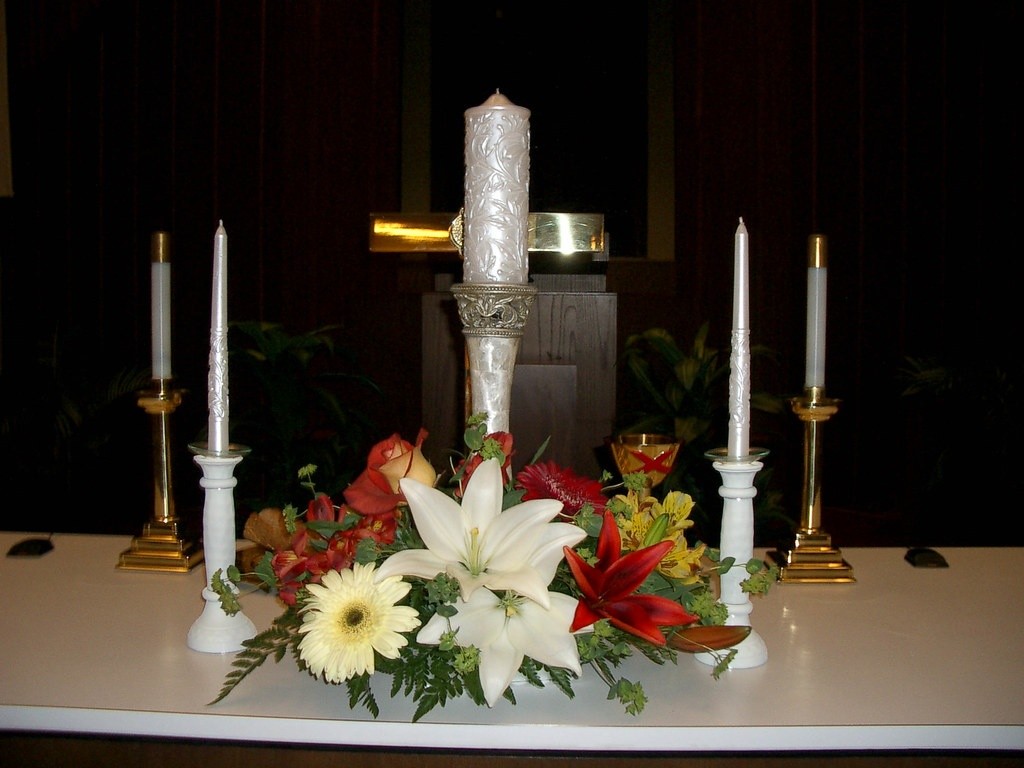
[208,413,782,724]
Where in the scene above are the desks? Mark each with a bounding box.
[0,532,1024,768]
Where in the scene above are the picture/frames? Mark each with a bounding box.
[399,2,678,266]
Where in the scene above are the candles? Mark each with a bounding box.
[206,219,230,456]
[805,232,826,406]
[728,218,753,459]
[464,88,530,285]
[151,232,172,381]
